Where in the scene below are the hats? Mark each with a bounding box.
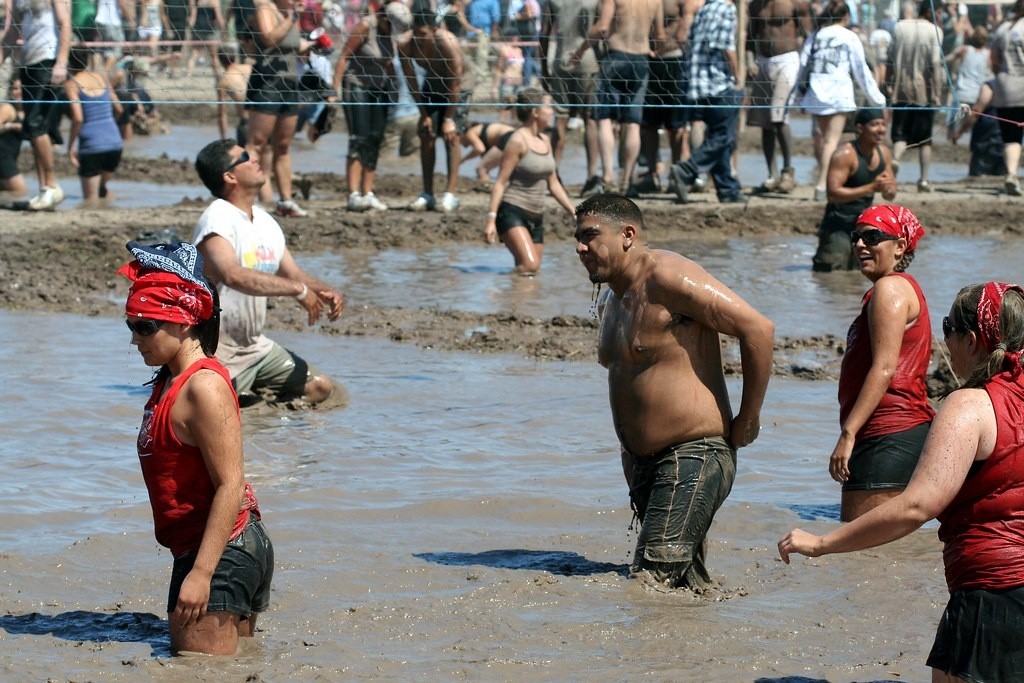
[117,238,214,325]
[854,106,884,124]
[384,1,414,30]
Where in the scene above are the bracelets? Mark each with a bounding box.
[294,279,314,310]
[485,210,498,219]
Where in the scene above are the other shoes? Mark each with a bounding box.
[275,199,307,217]
[433,191,461,213]
[346,191,388,211]
[26,183,64,211]
[670,163,688,203]
[917,179,934,192]
[408,192,435,211]
[721,190,750,202]
[1004,174,1023,196]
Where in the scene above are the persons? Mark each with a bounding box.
[0,0,1024,218]
[827,203,939,524]
[481,89,581,275]
[189,138,343,407]
[773,281,1024,682]
[811,106,897,276]
[113,237,274,656]
[574,193,776,591]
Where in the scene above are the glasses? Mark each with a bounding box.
[849,228,900,246]
[124,319,164,337]
[943,316,964,339]
[224,151,250,171]
